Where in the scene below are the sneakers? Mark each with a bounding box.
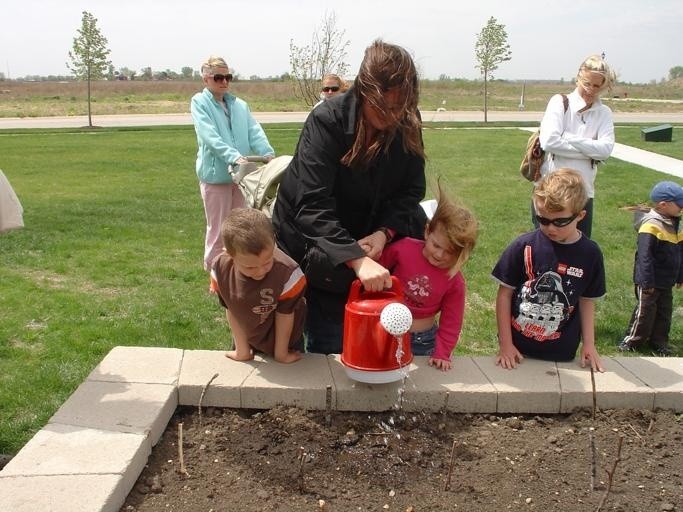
[617,341,672,355]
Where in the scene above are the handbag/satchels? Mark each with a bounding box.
[520,93,570,182]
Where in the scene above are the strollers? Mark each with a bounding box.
[225,154,300,220]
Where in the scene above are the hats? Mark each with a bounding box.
[651,180,683,209]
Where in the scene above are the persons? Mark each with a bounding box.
[362,176,477,373]
[529,55,614,240]
[188,57,276,269]
[313,73,349,110]
[272,41,427,356]
[212,207,307,363]
[492,168,607,374]
[619,181,683,354]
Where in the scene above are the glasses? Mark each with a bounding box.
[321,85,341,93]
[207,73,234,81]
[533,212,581,227]
